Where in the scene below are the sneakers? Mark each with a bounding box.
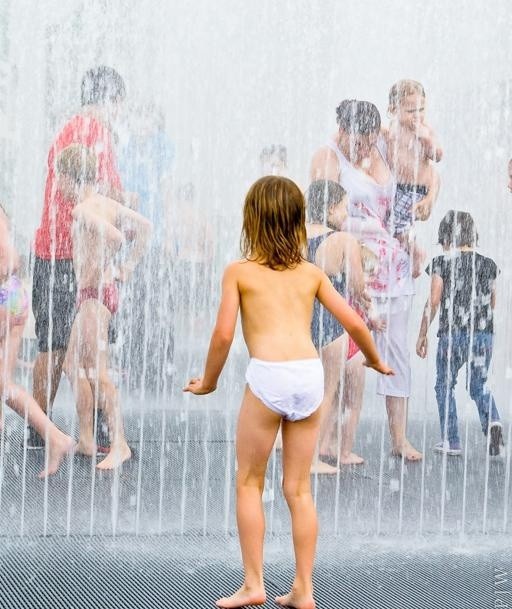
[432,438,463,457]
[486,419,506,458]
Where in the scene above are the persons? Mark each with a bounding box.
[180,175,393,609]
